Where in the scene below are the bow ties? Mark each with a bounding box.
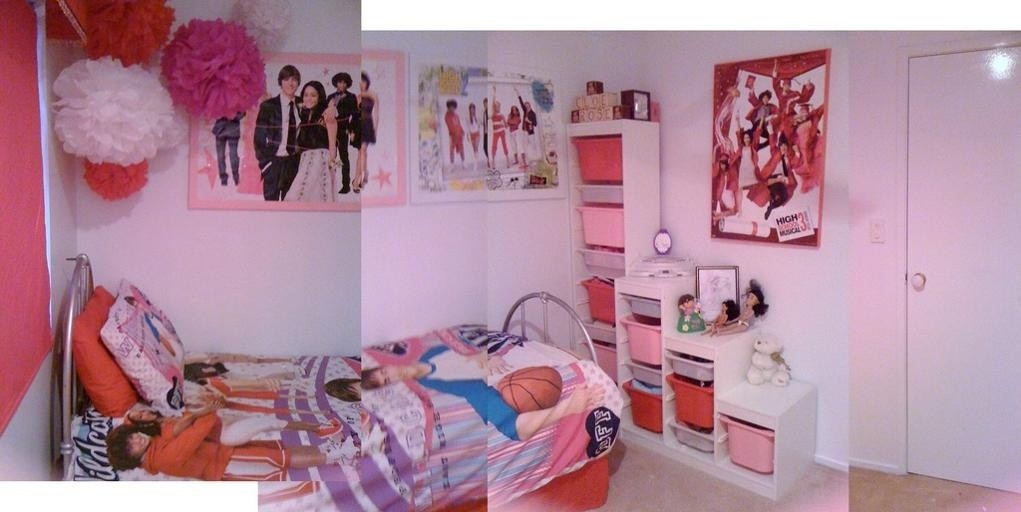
[335,91,342,96]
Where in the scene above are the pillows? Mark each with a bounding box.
[70,283,140,418]
[98,277,188,420]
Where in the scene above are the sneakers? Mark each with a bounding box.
[320,431,345,449]
[325,417,340,426]
[317,425,343,439]
[339,433,361,471]
[765,207,771,220]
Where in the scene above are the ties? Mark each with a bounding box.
[781,155,787,177]
[285,100,295,155]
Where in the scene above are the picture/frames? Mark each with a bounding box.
[707,46,831,251]
[694,265,741,327]
[407,56,567,202]
[186,49,415,213]
[653,228,672,256]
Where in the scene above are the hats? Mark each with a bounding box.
[775,77,801,85]
[332,73,352,87]
[796,104,813,113]
[759,90,771,100]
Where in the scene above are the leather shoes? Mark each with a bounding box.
[338,186,349,193]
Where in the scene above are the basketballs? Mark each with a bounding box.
[498,366,562,412]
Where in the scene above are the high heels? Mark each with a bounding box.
[351,179,359,193]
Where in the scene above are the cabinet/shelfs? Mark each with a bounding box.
[569,120,660,384]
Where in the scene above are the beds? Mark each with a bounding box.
[54,253,632,512]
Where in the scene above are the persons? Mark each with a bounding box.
[714,59,824,225]
[124,295,185,364]
[252,64,339,202]
[104,358,358,479]
[443,81,543,174]
[282,80,343,202]
[211,112,246,189]
[677,294,708,332]
[325,374,363,403]
[328,70,361,195]
[352,73,378,195]
[364,336,607,443]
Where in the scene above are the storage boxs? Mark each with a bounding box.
[622,380,661,435]
[572,136,623,182]
[578,206,623,251]
[575,184,625,207]
[716,414,775,477]
[667,374,713,432]
[579,277,616,327]
[617,318,661,366]
[664,352,713,383]
[626,359,661,388]
[582,341,617,379]
[622,294,660,321]
[668,418,713,455]
[582,250,624,274]
